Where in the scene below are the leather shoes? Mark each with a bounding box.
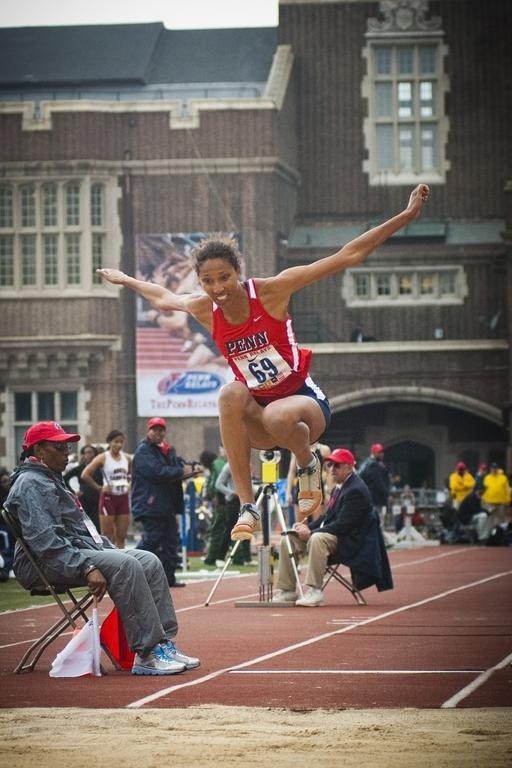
[173,583,185,587]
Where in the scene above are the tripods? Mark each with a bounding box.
[205,485,305,608]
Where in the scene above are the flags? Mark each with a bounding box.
[98,607,136,672]
[51,623,96,679]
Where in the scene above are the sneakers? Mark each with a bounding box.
[215,559,226,567]
[230,504,263,541]
[269,591,299,602]
[131,643,187,675]
[296,452,323,517]
[244,560,258,566]
[295,587,326,607]
[161,639,200,669]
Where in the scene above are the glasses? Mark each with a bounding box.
[328,463,340,468]
[44,443,73,452]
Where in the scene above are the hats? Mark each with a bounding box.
[323,448,355,465]
[491,463,499,468]
[22,421,80,451]
[456,462,466,470]
[147,418,166,432]
[480,463,488,469]
[371,444,383,453]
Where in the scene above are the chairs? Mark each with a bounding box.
[294,509,381,605]
[1,508,124,674]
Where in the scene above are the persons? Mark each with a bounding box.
[3,422,202,676]
[186,445,257,571]
[134,233,224,371]
[64,429,132,546]
[271,442,390,606]
[95,185,431,542]
[437,462,512,544]
